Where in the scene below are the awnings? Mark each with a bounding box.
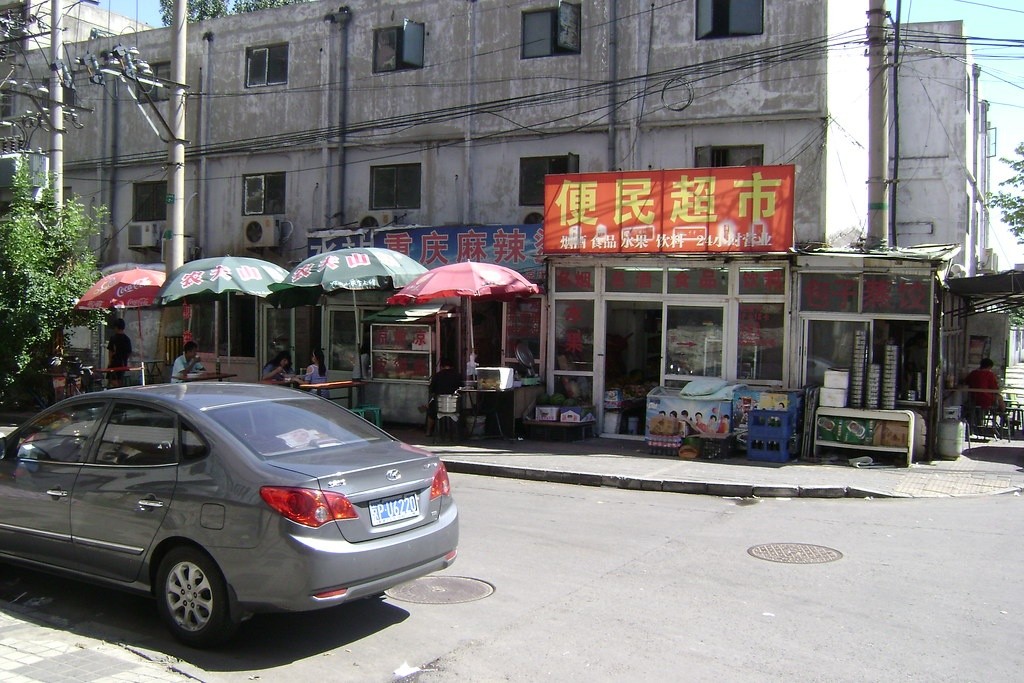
[942,269,1024,298]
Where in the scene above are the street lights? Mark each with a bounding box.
[49,0,102,220]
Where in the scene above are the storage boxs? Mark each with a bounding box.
[668,325,723,372]
[535,388,622,433]
[747,392,803,462]
[819,369,875,446]
[476,369,500,390]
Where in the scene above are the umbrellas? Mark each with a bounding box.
[386,259,541,379]
[159,257,290,382]
[267,248,430,377]
[74,268,167,386]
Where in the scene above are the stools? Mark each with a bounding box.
[438,413,460,442]
[960,400,1024,454]
[351,407,383,429]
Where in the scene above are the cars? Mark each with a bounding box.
[0,381,459,645]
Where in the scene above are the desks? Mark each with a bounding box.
[1003,385,1024,411]
[32,366,145,412]
[458,388,514,446]
[300,381,369,410]
[521,421,598,442]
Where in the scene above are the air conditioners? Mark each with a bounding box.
[519,207,545,225]
[358,210,393,228]
[981,247,999,274]
[126,220,196,263]
[243,215,279,247]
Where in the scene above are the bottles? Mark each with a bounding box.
[702,442,721,458]
[647,434,682,457]
[216,356,220,374]
[754,406,780,451]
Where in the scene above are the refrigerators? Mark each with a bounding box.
[645,384,754,440]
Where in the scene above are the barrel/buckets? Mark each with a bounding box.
[628,418,639,435]
[466,415,486,440]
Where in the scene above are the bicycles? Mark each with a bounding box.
[61,354,93,402]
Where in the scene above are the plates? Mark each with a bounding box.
[187,371,206,376]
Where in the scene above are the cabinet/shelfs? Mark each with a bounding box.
[704,327,763,381]
[370,322,432,382]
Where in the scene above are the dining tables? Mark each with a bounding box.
[171,371,305,391]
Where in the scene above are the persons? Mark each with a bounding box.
[753,399,757,410]
[352,344,369,409]
[669,410,678,418]
[107,318,132,390]
[171,341,207,384]
[681,410,694,421]
[707,415,717,433]
[736,398,743,408]
[964,358,1006,427]
[47,409,94,460]
[425,358,464,436]
[659,411,666,417]
[292,348,329,401]
[776,402,786,411]
[695,412,707,432]
[261,350,296,389]
[718,415,730,433]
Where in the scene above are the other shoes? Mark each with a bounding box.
[426,434,432,437]
[464,432,470,437]
[1009,420,1020,426]
